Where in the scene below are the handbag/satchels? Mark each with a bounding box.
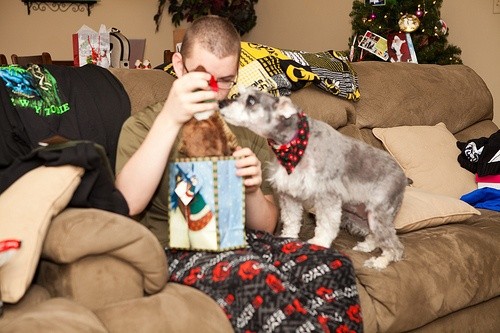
[109,27,130,69]
[72,24,110,68]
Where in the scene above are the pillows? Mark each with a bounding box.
[0,160,84,305]
[372,122,470,176]
[308,171,481,235]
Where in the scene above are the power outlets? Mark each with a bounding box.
[493,0,500,14]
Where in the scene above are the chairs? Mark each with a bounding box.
[10,52,51,69]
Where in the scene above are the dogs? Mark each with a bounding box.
[217,86,413,270]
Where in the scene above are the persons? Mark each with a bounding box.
[112,15,364,333]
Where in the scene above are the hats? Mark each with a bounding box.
[393,35,400,40]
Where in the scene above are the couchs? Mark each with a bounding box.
[0,54,500,333]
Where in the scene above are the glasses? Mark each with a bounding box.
[183,62,239,89]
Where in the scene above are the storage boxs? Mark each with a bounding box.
[169,156,250,254]
